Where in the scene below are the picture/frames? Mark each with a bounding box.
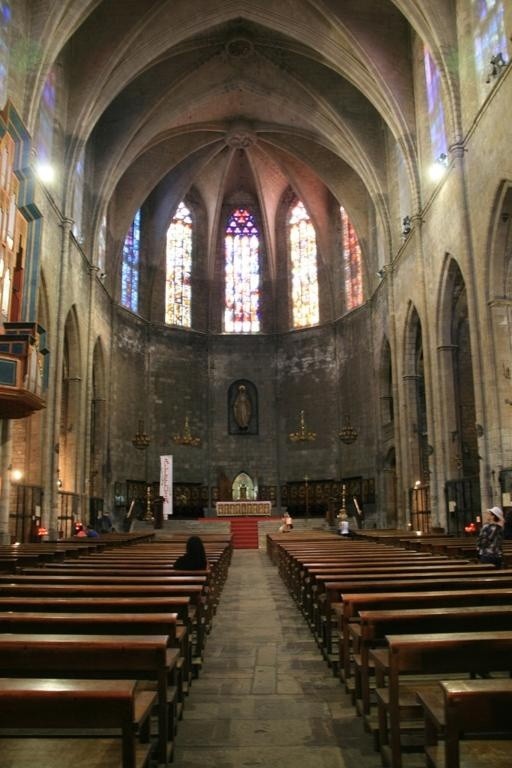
[215,500,272,518]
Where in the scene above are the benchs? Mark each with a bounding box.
[265,529,512,768]
[1,529,237,768]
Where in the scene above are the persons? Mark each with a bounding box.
[83,522,98,539]
[337,508,349,520]
[74,523,87,538]
[476,507,505,568]
[278,513,294,534]
[239,482,250,501]
[172,533,208,570]
[337,514,350,537]
[232,384,252,430]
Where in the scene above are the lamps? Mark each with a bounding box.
[172,416,201,446]
[130,418,152,451]
[336,411,358,446]
[288,410,317,442]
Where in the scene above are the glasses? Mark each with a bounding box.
[130,418,152,451]
[288,410,317,442]
[336,411,358,446]
[172,416,201,446]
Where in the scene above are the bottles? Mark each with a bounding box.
[487,506,506,522]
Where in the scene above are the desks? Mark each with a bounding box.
[215,500,272,518]
